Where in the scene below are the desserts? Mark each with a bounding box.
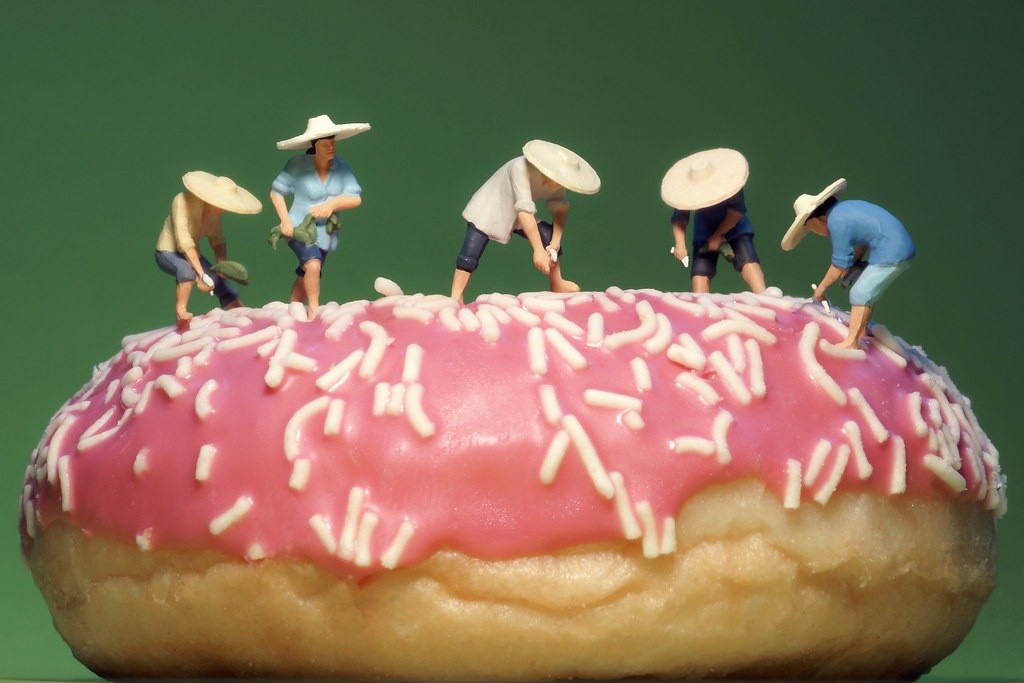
[19,277,1006,683]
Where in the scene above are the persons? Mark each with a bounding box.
[155,115,915,349]
